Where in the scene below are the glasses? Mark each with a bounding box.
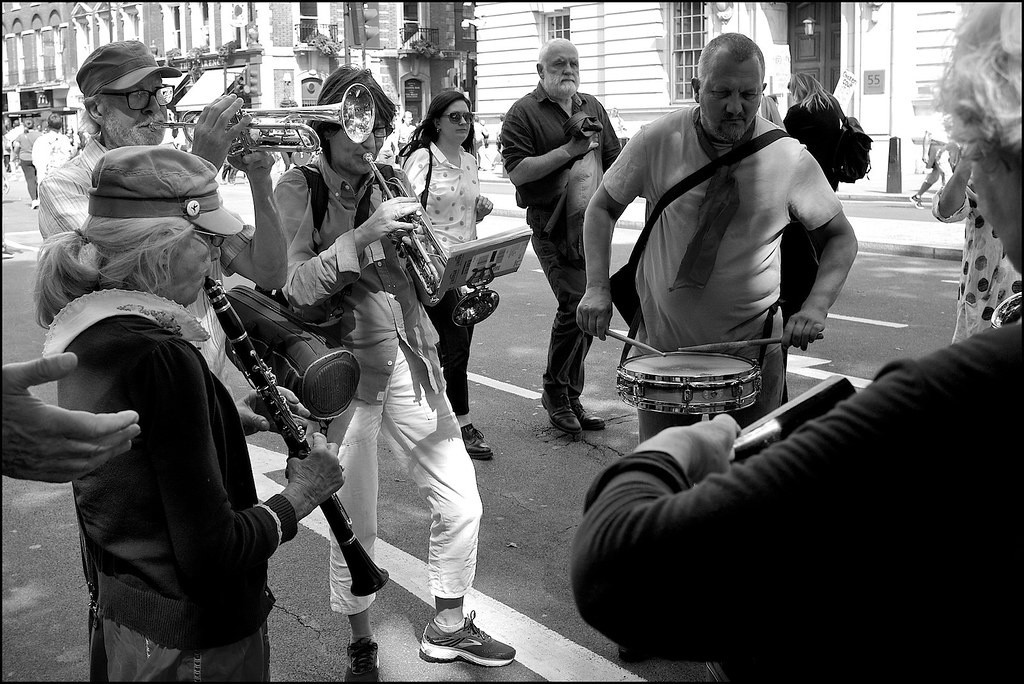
[174,227,227,248]
[94,83,175,110]
[371,123,393,138]
[440,112,475,125]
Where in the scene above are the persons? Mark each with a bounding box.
[501,38,624,436]
[928,144,1024,346]
[573,32,860,467]
[909,109,954,212]
[36,37,290,401]
[0,352,142,483]
[555,0,1024,684]
[31,140,346,684]
[401,92,495,462]
[0,114,80,260]
[273,66,517,684]
[785,74,842,223]
[403,91,495,461]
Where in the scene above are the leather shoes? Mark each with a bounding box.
[569,398,606,431]
[540,389,582,434]
[461,422,494,459]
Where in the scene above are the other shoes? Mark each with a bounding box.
[909,194,924,209]
[31,200,39,209]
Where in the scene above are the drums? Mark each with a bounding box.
[616,351,763,415]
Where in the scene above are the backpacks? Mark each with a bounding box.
[828,95,873,183]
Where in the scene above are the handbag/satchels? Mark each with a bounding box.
[609,205,822,332]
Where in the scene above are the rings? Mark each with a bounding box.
[485,204,490,208]
[338,463,346,472]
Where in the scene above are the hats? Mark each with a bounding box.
[76,39,181,98]
[88,145,244,236]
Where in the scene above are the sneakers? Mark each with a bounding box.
[419,610,517,667]
[344,637,379,683]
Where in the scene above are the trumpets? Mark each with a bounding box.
[362,152,499,326]
[148,83,376,158]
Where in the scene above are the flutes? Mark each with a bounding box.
[202,275,389,597]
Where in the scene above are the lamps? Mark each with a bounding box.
[802,1,815,36]
